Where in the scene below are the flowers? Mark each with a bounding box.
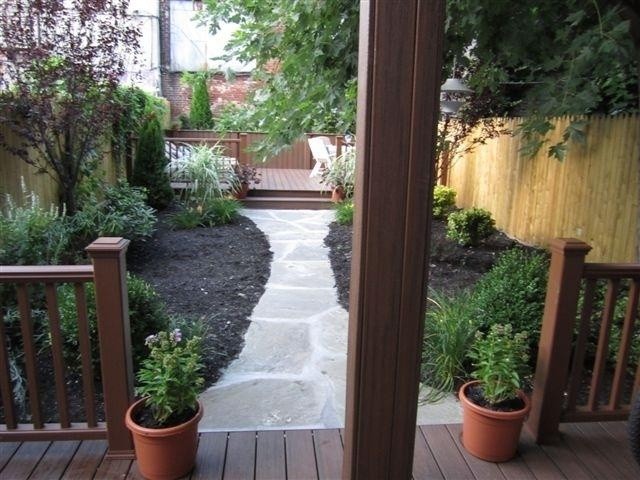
[466,323,530,405]
[138,327,206,425]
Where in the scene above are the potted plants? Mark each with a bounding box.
[227,162,263,199]
[318,164,346,202]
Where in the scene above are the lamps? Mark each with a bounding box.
[439,54,474,115]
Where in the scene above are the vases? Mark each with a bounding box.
[125,395,204,480]
[459,381,532,463]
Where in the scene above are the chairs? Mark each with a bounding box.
[307,138,335,177]
